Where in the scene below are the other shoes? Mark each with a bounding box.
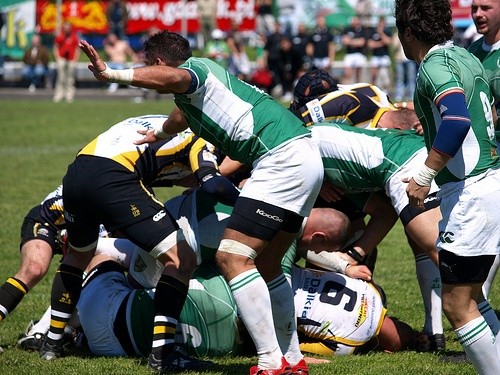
[39,338,74,360]
[415,330,446,355]
[16,319,44,351]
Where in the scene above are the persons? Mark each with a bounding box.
[24,35,49,91]
[466,0,500,299]
[249,17,335,101]
[341,15,367,84]
[367,16,393,89]
[205,29,249,81]
[395,0,500,375]
[77,30,324,375]
[103,32,136,90]
[393,32,417,102]
[53,24,79,103]
[0,67,446,361]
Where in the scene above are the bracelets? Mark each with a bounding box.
[108,68,134,85]
[155,125,170,139]
[347,248,363,263]
[412,164,438,186]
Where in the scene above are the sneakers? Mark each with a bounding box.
[249,357,292,375]
[147,348,216,373]
[291,360,309,375]
